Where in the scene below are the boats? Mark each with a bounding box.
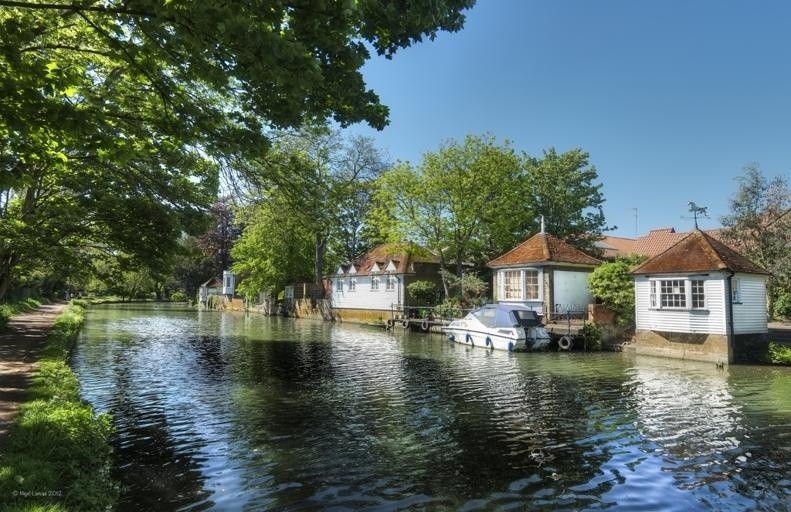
[441,304,552,352]
